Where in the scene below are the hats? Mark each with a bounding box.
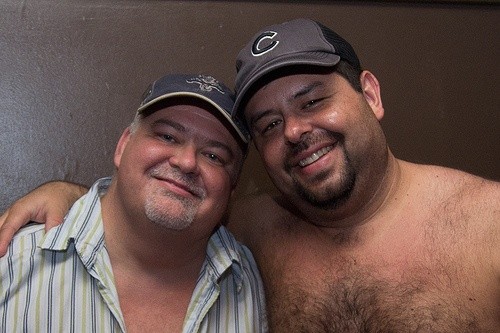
[137,73,252,146]
[230,17,363,122]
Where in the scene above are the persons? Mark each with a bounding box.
[0,16,500,333]
[0,71,269,333]
